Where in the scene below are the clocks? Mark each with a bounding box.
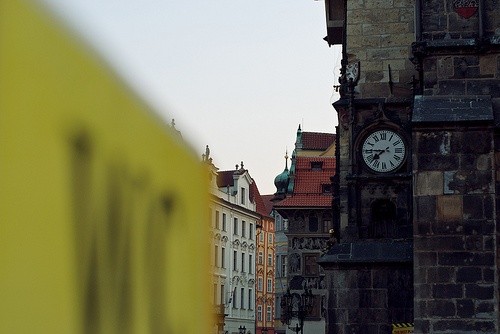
[355,121,413,174]
